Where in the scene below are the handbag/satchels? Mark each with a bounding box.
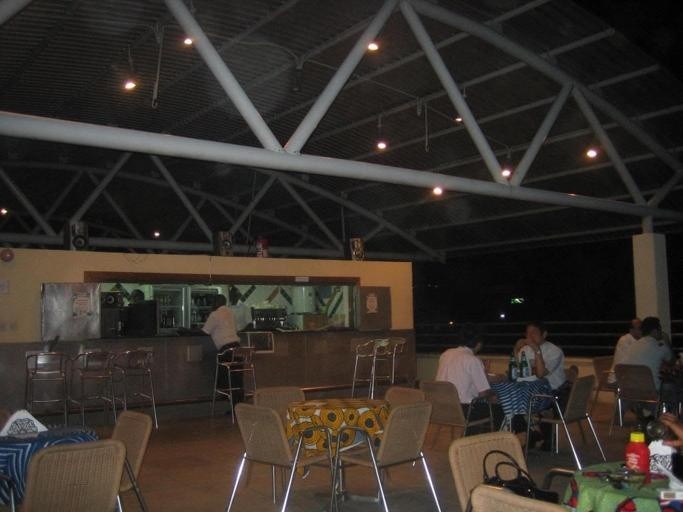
[464,449,560,512]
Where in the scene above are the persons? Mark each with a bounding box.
[513,321,566,412]
[434,332,545,450]
[199,294,244,414]
[606,318,641,389]
[227,287,253,333]
[620,317,673,394]
[659,412,683,448]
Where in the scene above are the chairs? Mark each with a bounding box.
[350,336,407,399]
[344,387,426,505]
[103,349,159,432]
[210,345,258,425]
[65,351,117,429]
[609,363,664,443]
[591,356,618,411]
[22,439,126,512]
[525,375,608,470]
[412,380,494,469]
[24,351,74,429]
[448,430,531,512]
[111,411,151,492]
[329,405,442,512]
[226,403,340,512]
[468,483,564,512]
[253,387,307,505]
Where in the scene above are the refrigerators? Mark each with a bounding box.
[187,286,218,330]
[144,285,186,338]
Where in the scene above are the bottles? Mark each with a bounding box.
[508,352,518,381]
[520,351,529,377]
[624,431,649,473]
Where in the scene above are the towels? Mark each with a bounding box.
[516,374,538,382]
[304,401,327,406]
